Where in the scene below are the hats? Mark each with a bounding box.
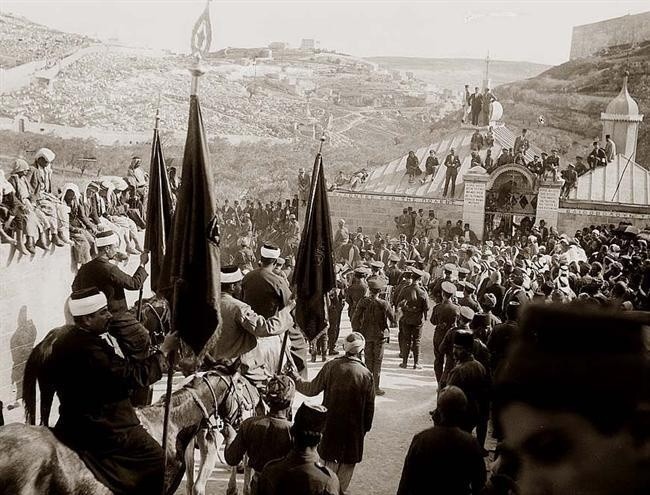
[95,229,118,247]
[459,306,474,320]
[338,258,477,298]
[491,305,649,441]
[295,400,328,433]
[244,201,296,269]
[220,265,245,284]
[485,239,649,295]
[263,375,295,411]
[68,286,108,316]
[438,387,467,420]
[502,128,611,170]
[473,311,492,329]
[453,328,474,348]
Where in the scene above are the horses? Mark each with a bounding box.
[22,292,178,426]
[0,352,247,495]
[184,332,298,493]
[221,226,298,268]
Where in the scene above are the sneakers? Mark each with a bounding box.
[307,349,339,363]
[398,351,422,368]
[375,388,385,396]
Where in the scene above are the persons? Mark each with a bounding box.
[0,149,649,494]
[587,135,616,172]
[460,86,530,155]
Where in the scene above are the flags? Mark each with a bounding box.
[143,99,223,363]
